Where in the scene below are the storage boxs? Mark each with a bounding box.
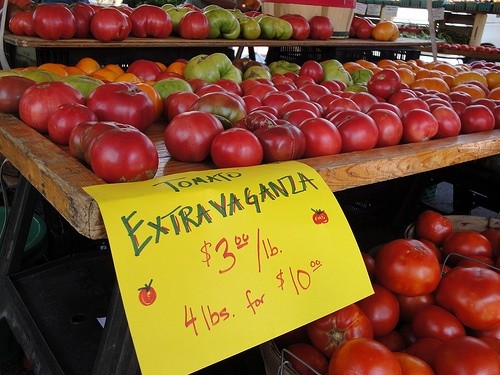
[358,0,500,14]
[273,46,421,65]
[435,12,487,47]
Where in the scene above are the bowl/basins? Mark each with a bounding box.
[261,0,357,39]
[0,206,46,253]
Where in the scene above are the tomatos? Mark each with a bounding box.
[0,0,399,45]
[0,50,500,182]
[280,210,500,375]
[435,44,500,53]
